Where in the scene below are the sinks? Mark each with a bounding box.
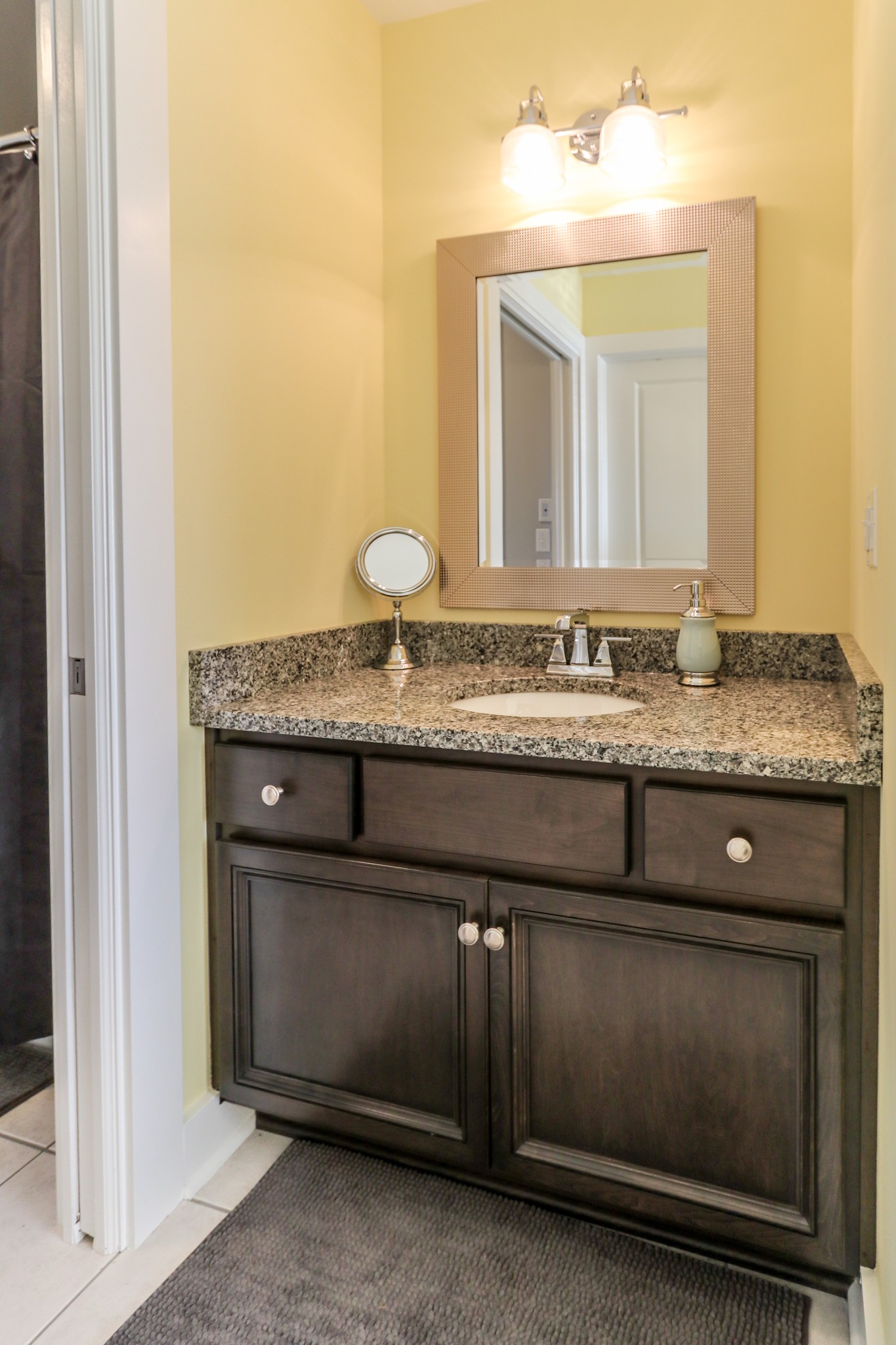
[437,675,656,718]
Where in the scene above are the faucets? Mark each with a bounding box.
[534,612,632,677]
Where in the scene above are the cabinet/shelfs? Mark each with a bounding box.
[202,727,880,1300]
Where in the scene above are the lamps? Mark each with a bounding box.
[499,66,688,202]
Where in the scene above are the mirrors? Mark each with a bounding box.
[436,195,756,614]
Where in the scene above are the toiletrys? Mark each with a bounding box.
[672,581,724,686]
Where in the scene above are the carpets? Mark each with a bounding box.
[0,1042,54,1120]
[104,1135,812,1345]
